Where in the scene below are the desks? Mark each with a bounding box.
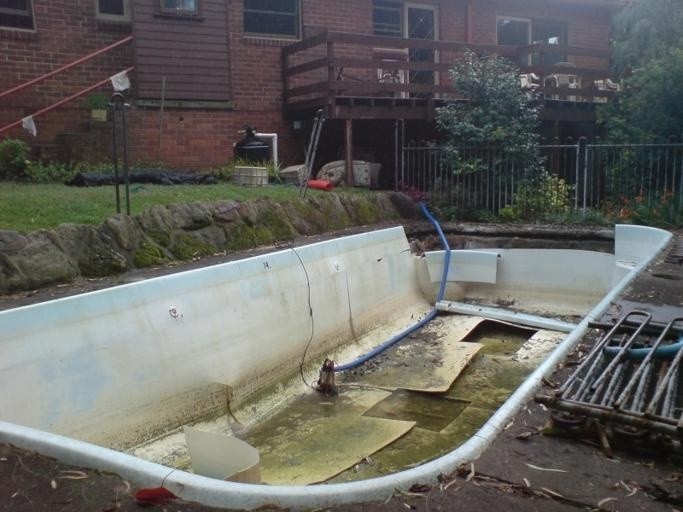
[543,74,582,100]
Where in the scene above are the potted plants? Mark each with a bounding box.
[87,92,113,123]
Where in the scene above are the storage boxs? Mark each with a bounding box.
[278,164,308,186]
[233,166,269,187]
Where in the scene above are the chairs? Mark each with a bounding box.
[593,78,620,103]
[519,74,532,88]
